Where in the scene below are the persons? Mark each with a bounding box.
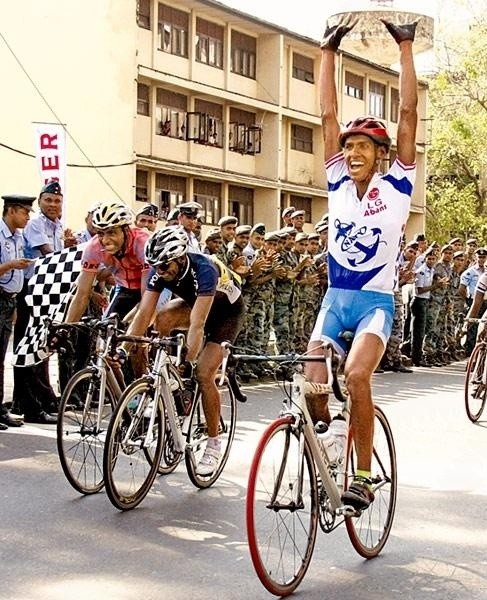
[106,224,245,477]
[180,126,186,140]
[245,143,253,154]
[162,121,171,136]
[303,12,424,509]
[209,133,217,144]
[229,132,235,151]
[0,182,487,432]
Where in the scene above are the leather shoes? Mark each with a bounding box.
[383,347,463,373]
[0,399,84,430]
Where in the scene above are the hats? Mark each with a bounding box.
[2,196,36,211]
[40,182,63,199]
[405,235,487,259]
[206,207,328,241]
[136,202,202,218]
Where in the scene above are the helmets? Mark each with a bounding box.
[92,203,132,230]
[144,225,189,266]
[338,116,390,154]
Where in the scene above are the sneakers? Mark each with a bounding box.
[341,482,374,508]
[195,447,222,475]
[238,364,277,379]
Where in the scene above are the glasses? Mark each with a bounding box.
[158,263,171,271]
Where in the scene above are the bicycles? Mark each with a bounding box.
[98,323,236,512]
[218,332,399,597]
[454,312,486,423]
[40,312,183,496]
